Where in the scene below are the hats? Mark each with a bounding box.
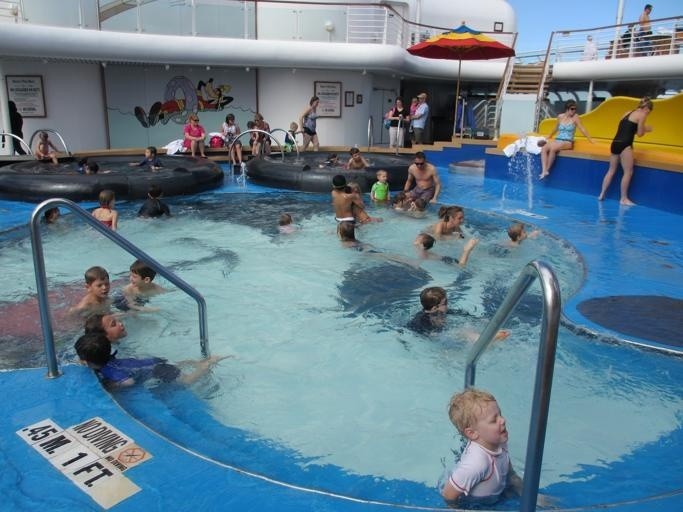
[417,93,426,97]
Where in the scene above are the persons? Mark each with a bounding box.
[387,97,409,148]
[403,151,441,206]
[536,99,596,181]
[250,112,272,156]
[183,114,208,159]
[345,184,383,224]
[77,156,90,174]
[285,122,302,153]
[504,223,541,248]
[348,172,363,196]
[338,220,378,254]
[597,97,655,207]
[404,287,510,344]
[130,146,165,173]
[221,113,243,167]
[137,184,173,220]
[581,35,601,64]
[414,233,480,268]
[247,121,262,157]
[318,152,346,170]
[85,161,99,176]
[2,101,27,155]
[346,147,375,170]
[299,97,320,152]
[406,93,429,145]
[85,312,135,360]
[541,98,556,119]
[67,266,118,320]
[371,169,391,202]
[90,190,118,233]
[330,174,366,222]
[410,98,419,133]
[111,258,174,313]
[621,23,639,54]
[393,192,413,214]
[275,211,302,235]
[74,332,235,389]
[412,198,428,219]
[638,3,655,58]
[427,205,468,241]
[36,131,62,166]
[44,206,72,225]
[441,389,551,509]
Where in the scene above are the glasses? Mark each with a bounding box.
[569,107,576,110]
[193,119,199,122]
[414,160,423,165]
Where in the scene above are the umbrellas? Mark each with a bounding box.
[405,21,515,137]
